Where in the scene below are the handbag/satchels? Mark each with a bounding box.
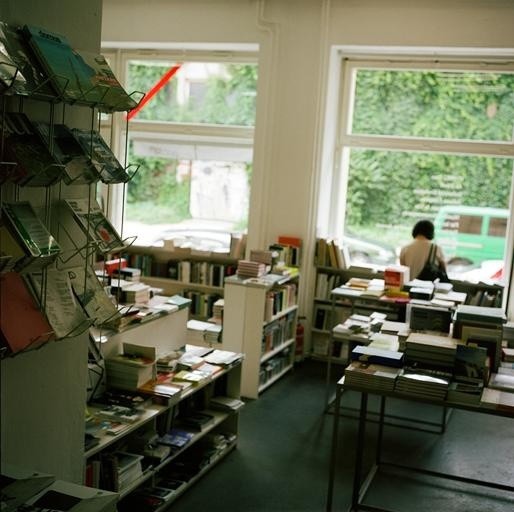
[418,264,450,282]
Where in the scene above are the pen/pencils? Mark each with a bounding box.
[201,349,215,357]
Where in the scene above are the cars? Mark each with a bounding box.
[446,258,503,286]
[146,220,244,251]
[338,223,398,266]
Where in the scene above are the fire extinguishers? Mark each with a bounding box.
[295,315,307,368]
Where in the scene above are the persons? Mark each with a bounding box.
[399,221,447,282]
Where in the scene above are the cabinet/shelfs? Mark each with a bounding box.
[83,343,242,512]
[222,275,301,399]
[309,267,504,361]
[0,62,145,361]
[124,244,238,320]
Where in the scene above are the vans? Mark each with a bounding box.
[431,204,510,268]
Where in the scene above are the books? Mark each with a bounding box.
[95,259,192,324]
[310,238,352,360]
[126,440,171,464]
[258,284,299,386]
[21,266,122,340]
[66,196,128,253]
[211,394,245,413]
[236,235,303,286]
[489,347,514,390]
[183,289,225,341]
[337,278,507,403]
[97,449,144,493]
[0,122,132,184]
[349,261,394,276]
[85,344,243,408]
[0,201,64,260]
[1,23,137,109]
[157,429,195,446]
[127,252,235,287]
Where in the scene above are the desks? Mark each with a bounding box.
[320,316,514,512]
